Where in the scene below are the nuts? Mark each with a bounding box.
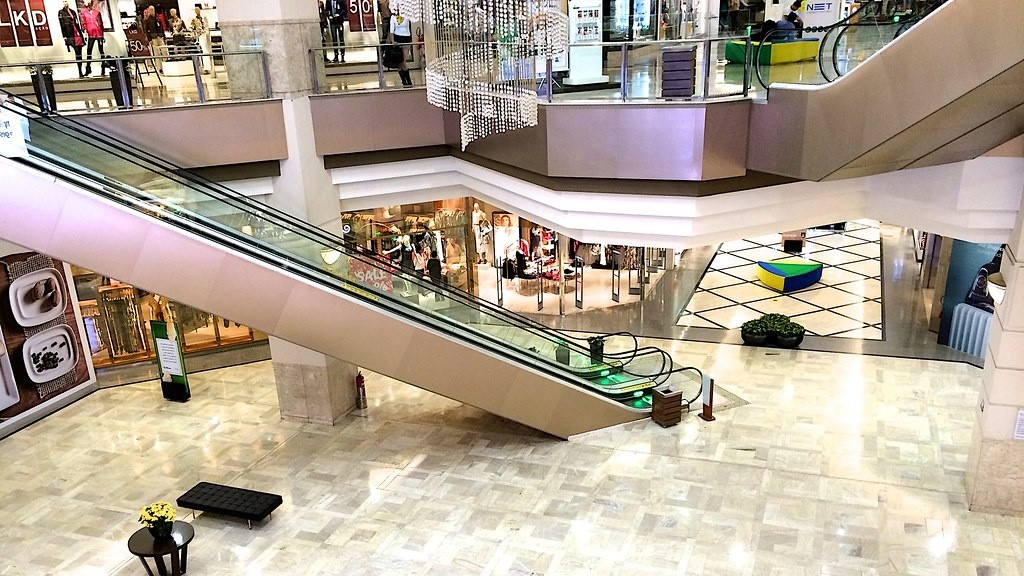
[32,277,60,306]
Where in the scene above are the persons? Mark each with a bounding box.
[446,239,464,283]
[719,0,733,36]
[318,0,348,63]
[472,203,485,256]
[476,219,493,264]
[58,0,105,79]
[382,235,423,294]
[740,1,803,42]
[143,6,214,75]
[494,215,519,260]
[390,9,412,88]
[427,251,445,302]
[414,245,427,296]
[377,0,392,57]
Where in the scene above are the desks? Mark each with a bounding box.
[128,520,194,576]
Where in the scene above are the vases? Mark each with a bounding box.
[148,523,173,539]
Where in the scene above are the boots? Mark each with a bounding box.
[398,67,413,85]
[83,66,91,76]
[100,65,106,75]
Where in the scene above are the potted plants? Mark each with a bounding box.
[741,313,805,348]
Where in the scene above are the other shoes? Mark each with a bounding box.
[160,69,163,73]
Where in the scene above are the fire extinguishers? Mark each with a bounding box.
[355,371,366,398]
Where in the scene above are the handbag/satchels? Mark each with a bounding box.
[382,45,404,68]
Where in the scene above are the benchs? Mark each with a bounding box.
[176,482,283,530]
[725,38,820,66]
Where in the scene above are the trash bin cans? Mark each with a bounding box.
[662,46,697,97]
[590,341,604,364]
[844,3,861,32]
[652,384,683,428]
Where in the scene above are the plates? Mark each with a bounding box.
[9,268,67,327]
[22,324,80,383]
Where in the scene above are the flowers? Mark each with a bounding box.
[137,501,176,528]
